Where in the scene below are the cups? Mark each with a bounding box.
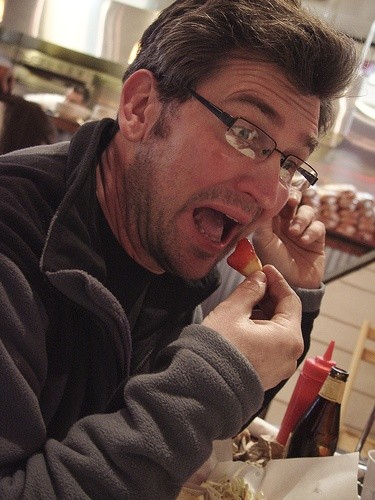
[359,450,375,500]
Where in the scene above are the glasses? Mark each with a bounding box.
[190,87,318,192]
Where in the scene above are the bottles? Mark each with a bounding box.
[283,365,350,459]
[273,338,336,447]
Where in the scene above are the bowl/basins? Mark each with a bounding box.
[56,102,90,123]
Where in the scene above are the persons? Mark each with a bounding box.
[0,0,356,500]
[0,61,90,157]
